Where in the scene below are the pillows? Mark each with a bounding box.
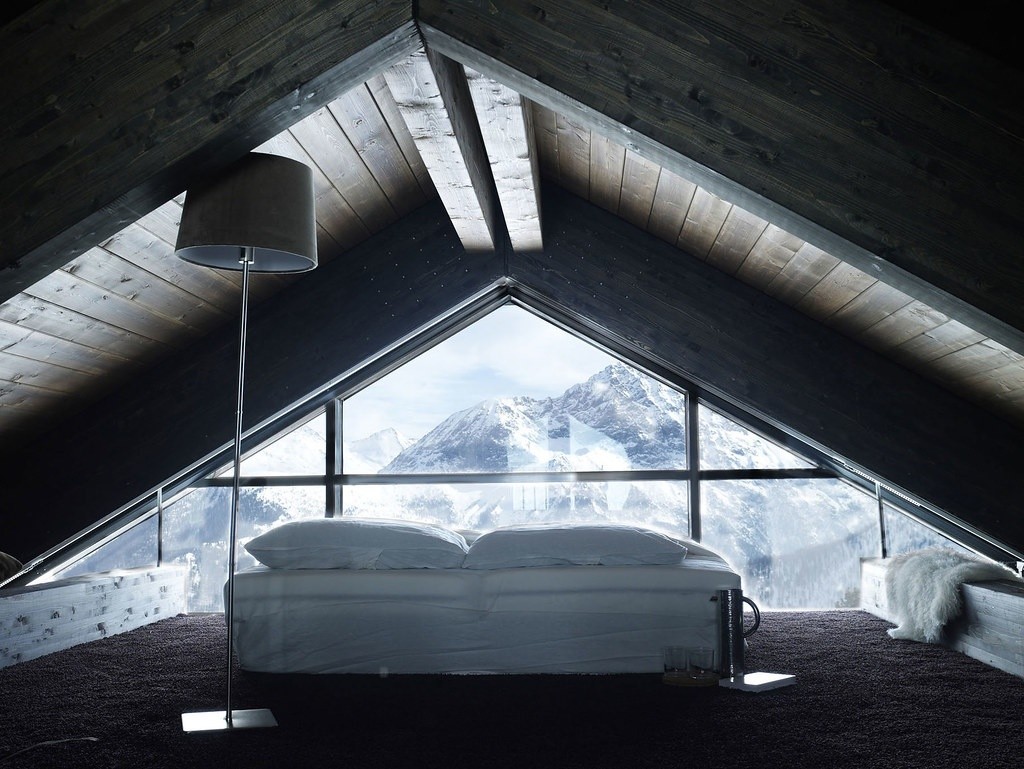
[244,517,469,569]
[463,523,689,569]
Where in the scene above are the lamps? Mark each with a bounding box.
[173,150,317,732]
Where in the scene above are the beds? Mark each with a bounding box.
[224,555,741,675]
